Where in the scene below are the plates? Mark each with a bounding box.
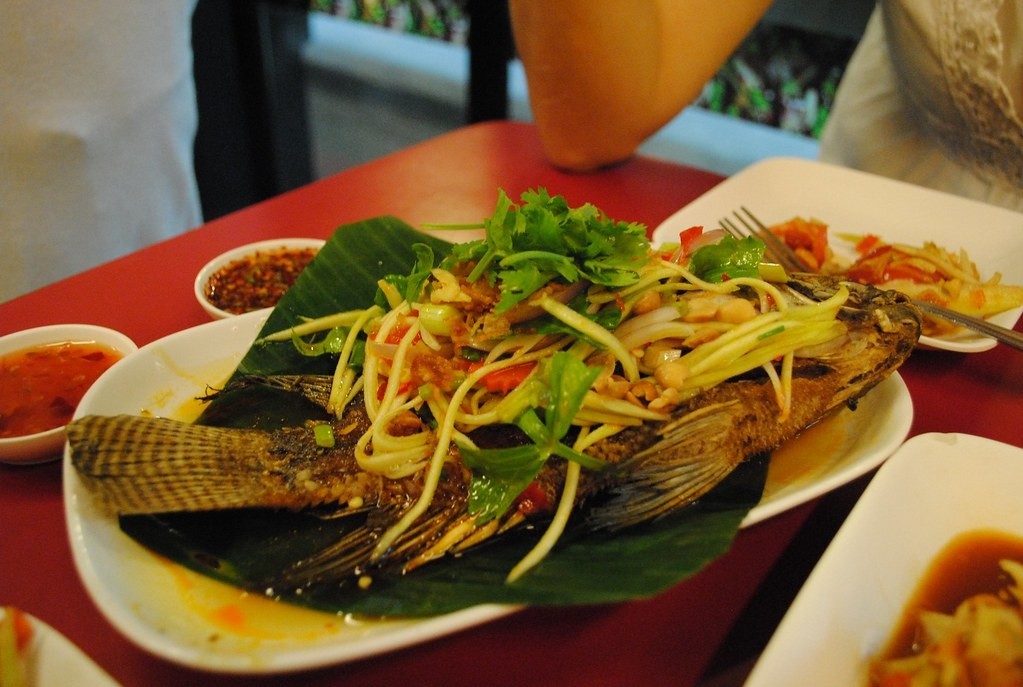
[0,607,123,687]
[64,308,912,673]
[652,158,1023,353]
[743,433,1023,687]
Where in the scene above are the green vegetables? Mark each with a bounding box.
[289,186,766,520]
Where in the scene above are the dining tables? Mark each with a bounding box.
[0,118,1023,687]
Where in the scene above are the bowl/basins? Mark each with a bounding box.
[0,324,137,464]
[196,238,326,321]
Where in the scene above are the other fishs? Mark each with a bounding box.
[65,275,921,585]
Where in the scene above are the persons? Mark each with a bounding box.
[1,1,319,309]
[507,0,1023,212]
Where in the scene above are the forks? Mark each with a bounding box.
[719,206,1023,350]
